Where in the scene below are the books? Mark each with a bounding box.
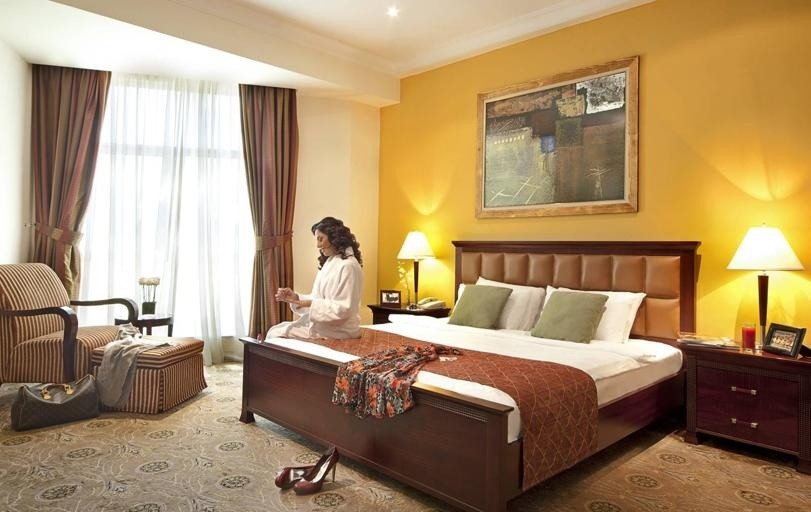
[676,330,740,350]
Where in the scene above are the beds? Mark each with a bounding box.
[239,239,701,511]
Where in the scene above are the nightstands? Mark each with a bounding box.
[365,303,451,325]
[674,337,811,471]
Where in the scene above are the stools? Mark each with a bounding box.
[92,336,208,415]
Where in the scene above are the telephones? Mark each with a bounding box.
[416,297,446,310]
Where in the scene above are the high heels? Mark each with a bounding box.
[275,447,339,495]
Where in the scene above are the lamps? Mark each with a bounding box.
[725,225,805,353]
[396,228,437,311]
[137,275,162,315]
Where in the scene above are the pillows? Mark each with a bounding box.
[447,275,647,345]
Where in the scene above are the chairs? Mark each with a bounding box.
[0,262,140,385]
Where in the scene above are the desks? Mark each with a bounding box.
[114,316,173,337]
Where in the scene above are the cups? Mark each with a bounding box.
[742,325,757,349]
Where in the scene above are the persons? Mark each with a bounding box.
[265,217,364,339]
[771,334,794,348]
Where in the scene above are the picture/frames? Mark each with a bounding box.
[760,321,804,356]
[379,287,402,308]
[472,53,639,221]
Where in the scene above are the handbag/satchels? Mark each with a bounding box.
[11,374,100,432]
